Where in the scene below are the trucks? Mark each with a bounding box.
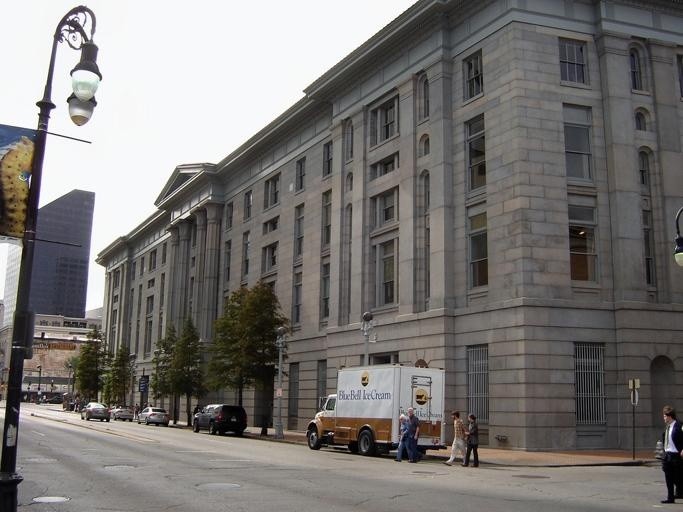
[305,362,446,456]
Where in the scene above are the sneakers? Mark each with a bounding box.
[661,495,683,503]
[442,460,478,468]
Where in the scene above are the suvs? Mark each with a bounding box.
[191,403,247,435]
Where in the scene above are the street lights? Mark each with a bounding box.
[2,3,98,512]
[153,349,158,408]
[128,353,135,407]
[37,366,40,400]
[274,327,286,439]
[50,380,53,393]
[67,365,73,393]
[361,311,373,367]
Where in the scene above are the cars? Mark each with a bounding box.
[81,402,110,423]
[107,405,133,422]
[137,406,170,426]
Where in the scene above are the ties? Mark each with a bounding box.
[667,425,670,445]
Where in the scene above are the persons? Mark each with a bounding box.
[394,414,417,463]
[407,408,419,459]
[193,405,201,415]
[443,410,469,466]
[660,406,683,503]
[460,413,479,467]
[133,401,152,420]
